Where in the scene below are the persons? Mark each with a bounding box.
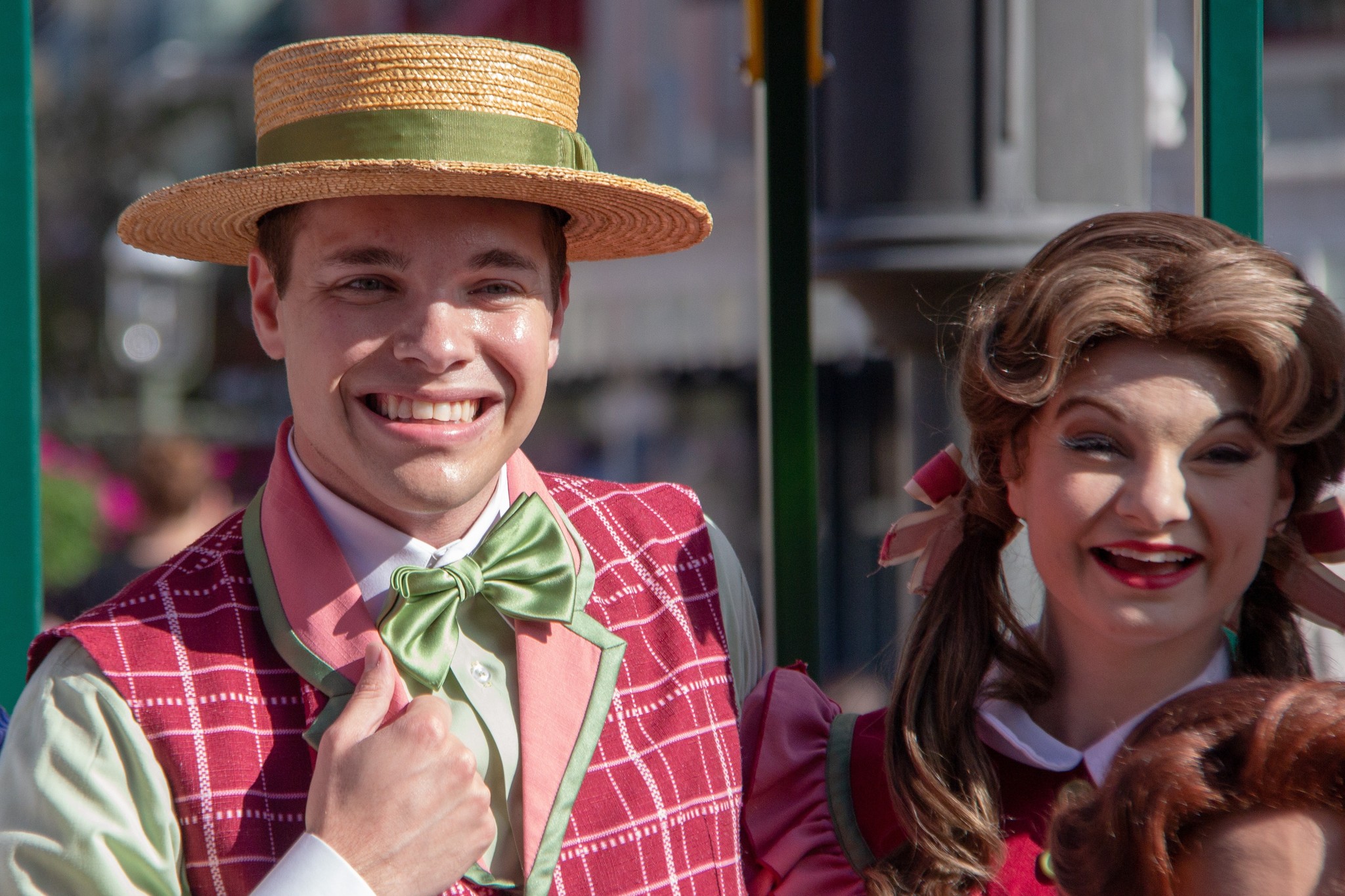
[45,439,248,625]
[1054,679,1345,896]
[1,32,768,896]
[736,213,1345,896]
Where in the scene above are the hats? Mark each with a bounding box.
[116,34,714,266]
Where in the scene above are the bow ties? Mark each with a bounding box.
[375,492,577,691]
